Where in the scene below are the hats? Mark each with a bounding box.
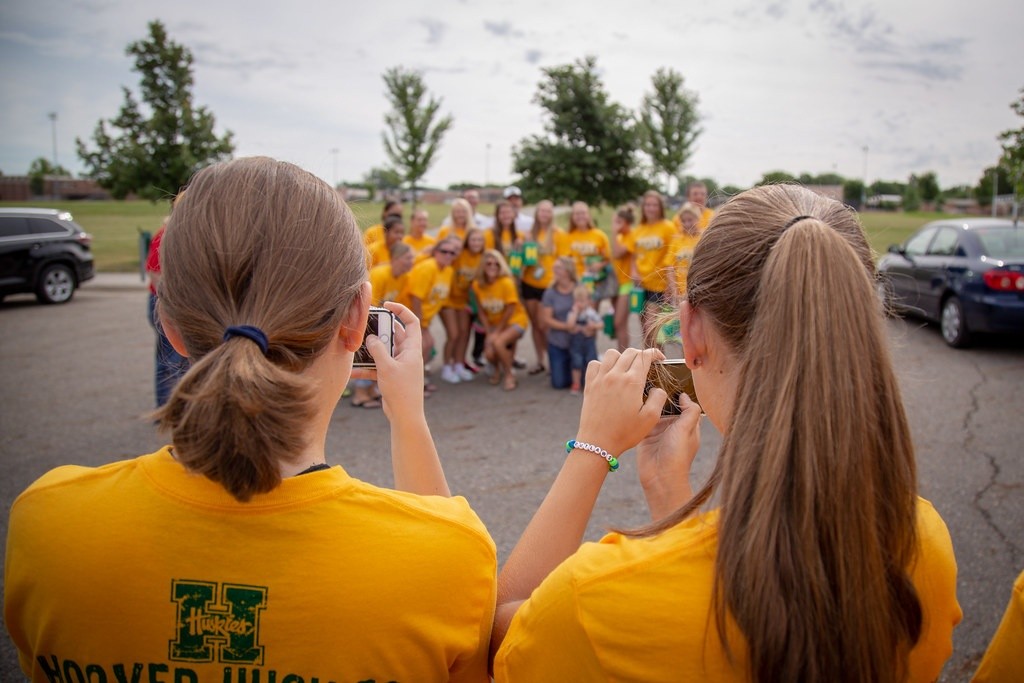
[503,186,522,198]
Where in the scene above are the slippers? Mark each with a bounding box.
[351,398,381,408]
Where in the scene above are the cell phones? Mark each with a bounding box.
[643,359,707,416]
[353,308,395,369]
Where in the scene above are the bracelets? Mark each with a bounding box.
[565,441,620,472]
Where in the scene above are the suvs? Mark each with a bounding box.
[0,205,97,307]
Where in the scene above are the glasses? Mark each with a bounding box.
[437,249,455,255]
[485,262,499,266]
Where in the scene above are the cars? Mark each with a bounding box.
[872,217,1024,347]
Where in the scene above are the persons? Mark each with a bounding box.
[147,183,717,423]
[3,158,500,683]
[483,185,1024,683]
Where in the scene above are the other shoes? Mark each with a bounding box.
[441,370,460,383]
[571,383,580,395]
[529,365,545,374]
[455,368,474,382]
[490,371,503,384]
[503,376,516,390]
